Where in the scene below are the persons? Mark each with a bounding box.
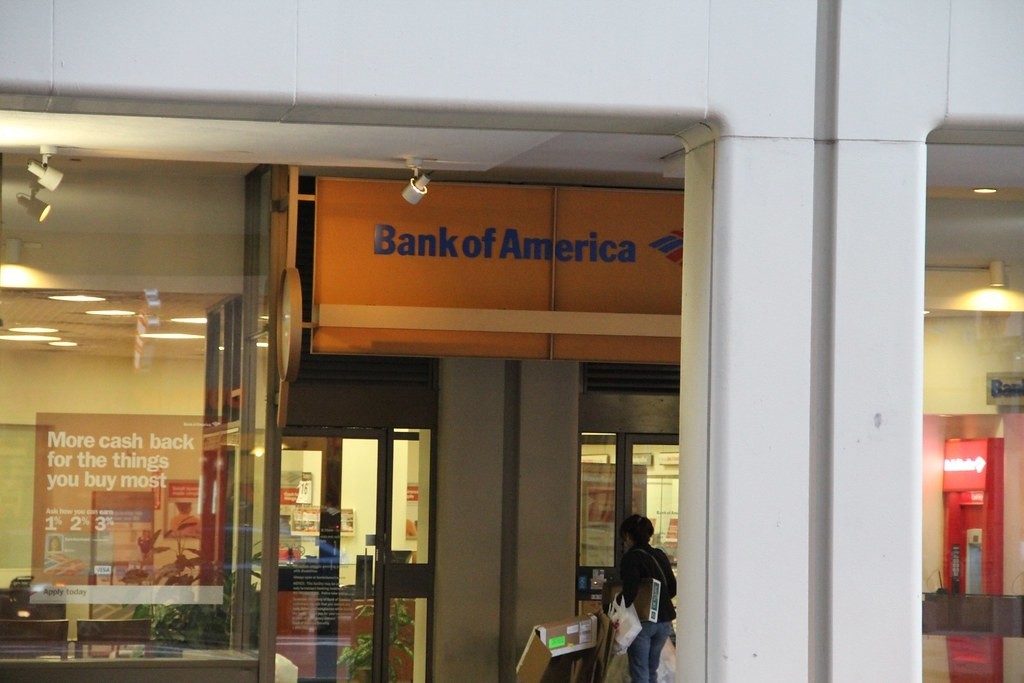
[48,536,61,551]
[616,514,677,683]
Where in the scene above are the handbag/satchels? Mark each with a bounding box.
[610,592,643,647]
[607,592,628,655]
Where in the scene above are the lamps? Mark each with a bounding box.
[27,145,65,193]
[401,170,433,205]
[16,181,51,223]
[988,261,1008,288]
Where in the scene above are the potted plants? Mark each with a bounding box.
[335,594,417,682]
